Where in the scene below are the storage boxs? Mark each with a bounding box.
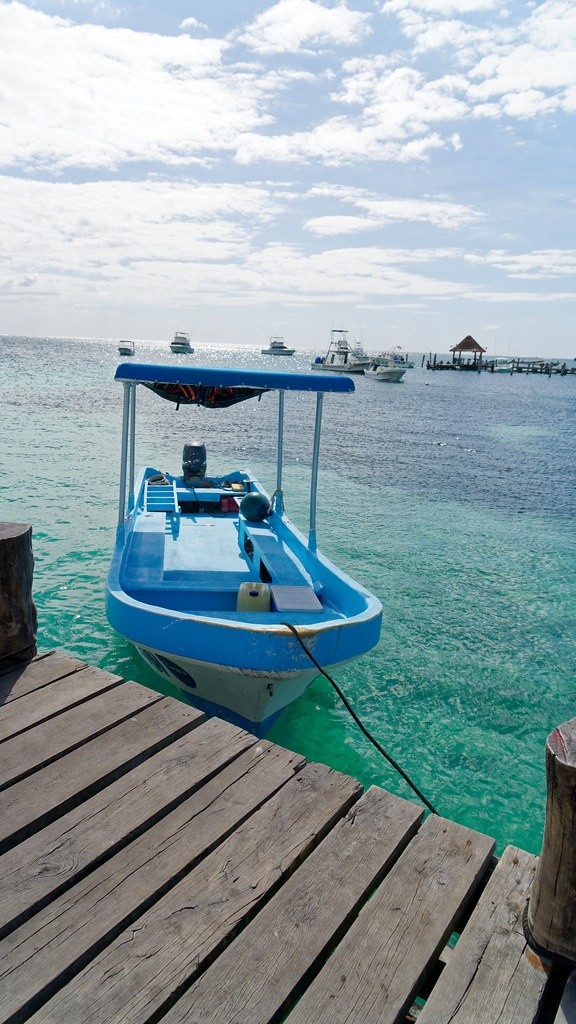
[270,585,324,614]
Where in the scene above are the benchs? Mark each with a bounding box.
[177,487,248,502]
[237,513,323,594]
[126,511,166,582]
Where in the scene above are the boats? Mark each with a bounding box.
[372,346,416,369]
[351,341,369,362]
[311,329,370,372]
[362,357,406,383]
[261,336,295,356]
[116,339,136,356]
[486,359,518,371]
[169,332,194,354]
[100,362,384,741]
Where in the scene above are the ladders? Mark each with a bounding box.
[143,479,180,515]
